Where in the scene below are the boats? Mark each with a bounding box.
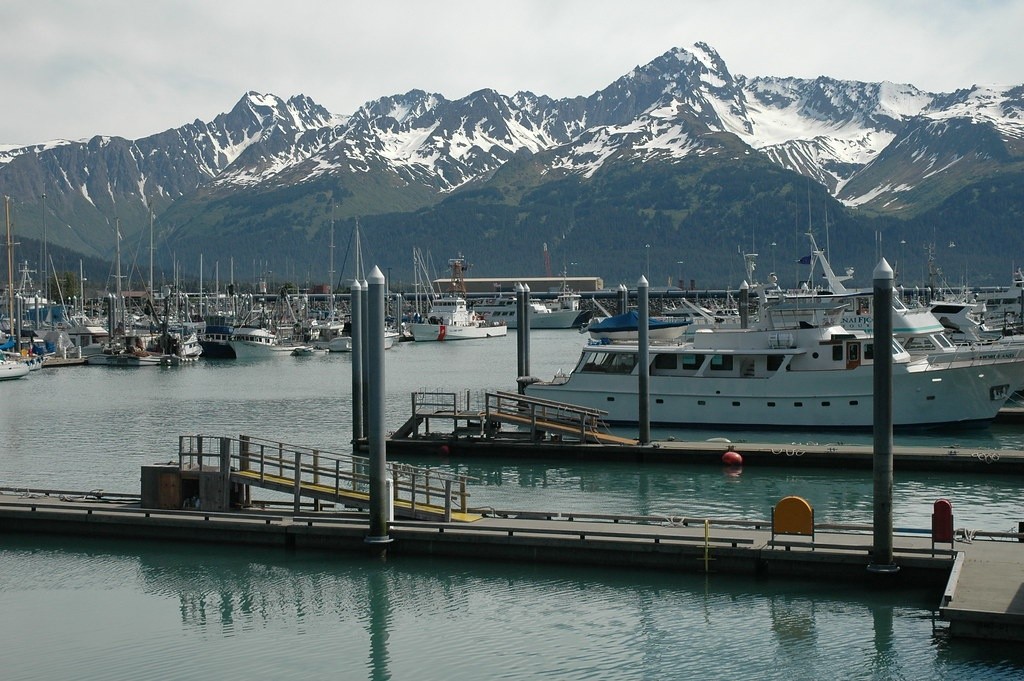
[579,311,693,342]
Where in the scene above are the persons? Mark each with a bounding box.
[1015,267,1023,286]
[801,281,807,294]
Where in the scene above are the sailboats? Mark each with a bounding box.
[0,180,1023,428]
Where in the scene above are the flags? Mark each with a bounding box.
[793,255,812,264]
